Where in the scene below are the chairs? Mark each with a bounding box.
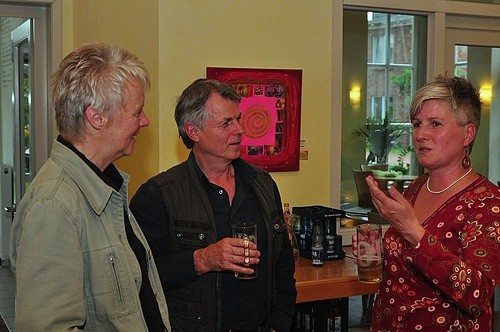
[352,164,389,225]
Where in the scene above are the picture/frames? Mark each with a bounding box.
[206,66,303,172]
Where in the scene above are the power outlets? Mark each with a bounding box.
[300,139,309,160]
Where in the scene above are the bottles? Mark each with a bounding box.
[302,297,342,332]
[283,202,335,267]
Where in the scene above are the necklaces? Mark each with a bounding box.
[426,166,473,194]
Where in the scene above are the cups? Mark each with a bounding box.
[232,222,257,279]
[356,223,384,284]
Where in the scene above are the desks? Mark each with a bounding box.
[375,174,420,193]
[293,244,383,332]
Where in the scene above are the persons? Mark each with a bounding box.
[129,79,298,332]
[351,72,500,332]
[9,41,173,332]
